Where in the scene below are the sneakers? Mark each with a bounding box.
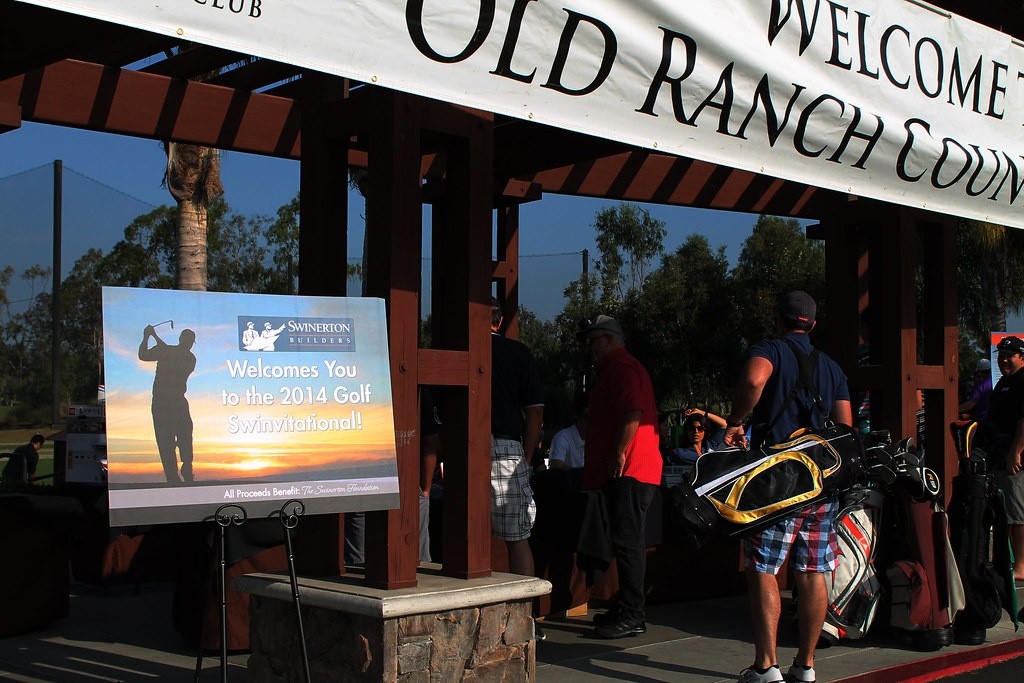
[736,665,785,683]
[593,608,646,638]
[785,664,816,683]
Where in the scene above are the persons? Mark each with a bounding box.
[139,321,197,486]
[340,293,1024,639]
[1,435,45,486]
[721,291,853,683]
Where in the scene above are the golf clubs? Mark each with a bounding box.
[828,428,941,505]
[949,420,995,486]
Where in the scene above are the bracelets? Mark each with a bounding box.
[704,411,709,418]
[725,415,743,427]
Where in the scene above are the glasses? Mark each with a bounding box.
[586,326,621,336]
[689,425,705,432]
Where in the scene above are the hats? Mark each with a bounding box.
[775,290,817,323]
[994,336,1024,355]
[491,295,500,307]
[975,358,991,371]
[33,435,44,443]
